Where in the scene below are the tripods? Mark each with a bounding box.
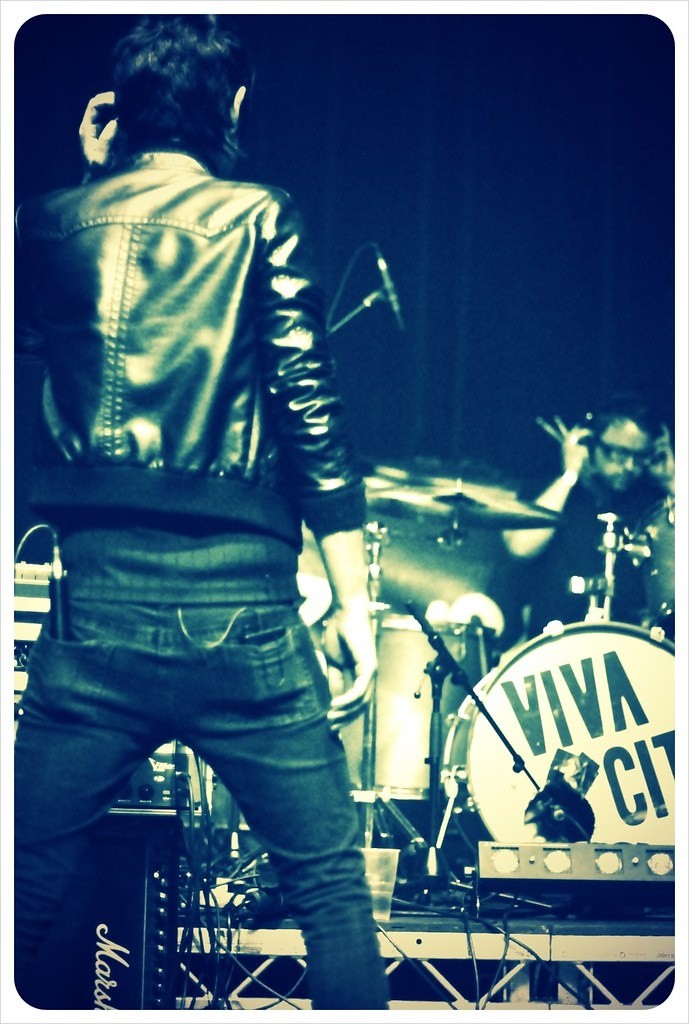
[208,514,567,921]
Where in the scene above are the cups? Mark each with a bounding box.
[358,847,400,921]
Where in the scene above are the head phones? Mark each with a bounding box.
[581,396,667,466]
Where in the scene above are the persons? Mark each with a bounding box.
[499,404,675,639]
[14,14,391,1010]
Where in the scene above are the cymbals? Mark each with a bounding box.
[369,479,565,531]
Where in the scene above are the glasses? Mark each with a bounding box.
[598,437,653,468]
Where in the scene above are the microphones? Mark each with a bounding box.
[543,799,565,821]
[375,249,405,331]
[50,537,70,643]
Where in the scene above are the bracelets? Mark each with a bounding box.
[560,468,577,484]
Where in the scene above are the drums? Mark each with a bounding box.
[436,618,676,851]
[332,605,498,804]
[630,494,675,618]
